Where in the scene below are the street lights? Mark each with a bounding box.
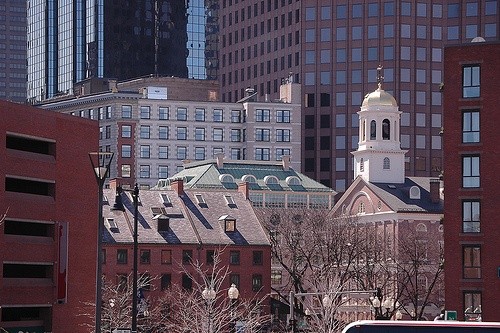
[87,149,115,332]
[116,181,140,333]
[201,284,217,333]
[227,282,240,333]
